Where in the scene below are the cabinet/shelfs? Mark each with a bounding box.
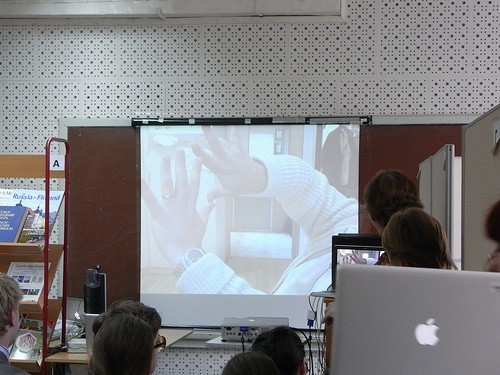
[0,152,62,375]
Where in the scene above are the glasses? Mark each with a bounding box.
[153,334,166,349]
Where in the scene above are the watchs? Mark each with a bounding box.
[175,248,204,278]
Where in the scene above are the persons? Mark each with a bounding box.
[0,273,30,375]
[222,326,306,375]
[88,300,161,375]
[143,123,359,294]
[483,199,500,272]
[383,207,448,269]
[337,250,377,265]
[364,169,423,234]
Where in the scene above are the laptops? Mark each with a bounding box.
[328,265,500,375]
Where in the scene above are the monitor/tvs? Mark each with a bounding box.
[331,245,385,265]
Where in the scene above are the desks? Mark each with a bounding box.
[207,335,326,353]
[45,326,193,375]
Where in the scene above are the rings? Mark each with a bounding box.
[162,193,174,198]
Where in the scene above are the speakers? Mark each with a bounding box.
[81,267,107,358]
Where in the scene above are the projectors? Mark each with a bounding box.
[221,317,289,343]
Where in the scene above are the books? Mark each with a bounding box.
[0,206,28,243]
